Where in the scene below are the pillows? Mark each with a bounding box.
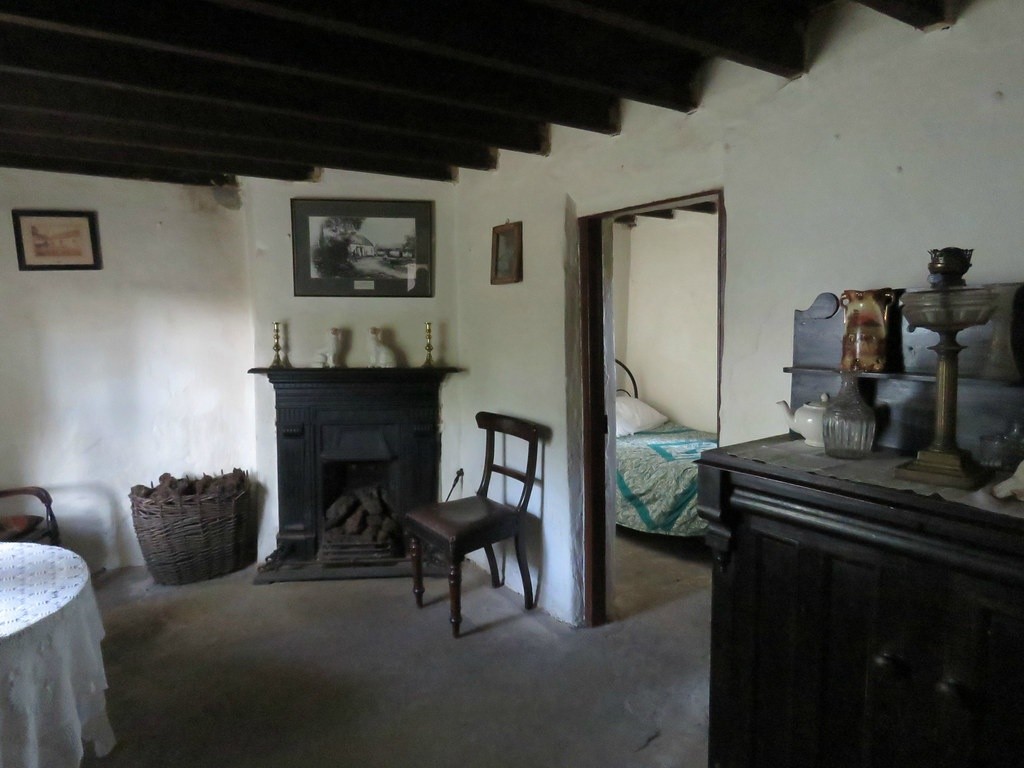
[616,396,668,438]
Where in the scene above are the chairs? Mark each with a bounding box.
[404,411,539,640]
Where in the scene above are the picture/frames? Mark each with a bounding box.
[11,208,103,271]
[290,198,433,298]
[491,221,522,285]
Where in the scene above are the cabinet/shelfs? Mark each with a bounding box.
[691,435,1024,768]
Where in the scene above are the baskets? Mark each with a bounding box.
[129,467,251,587]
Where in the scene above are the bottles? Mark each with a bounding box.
[821,368,877,461]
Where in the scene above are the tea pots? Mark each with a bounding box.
[776,392,835,447]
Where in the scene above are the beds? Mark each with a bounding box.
[614,359,717,536]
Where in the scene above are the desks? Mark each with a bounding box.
[0,542,118,768]
